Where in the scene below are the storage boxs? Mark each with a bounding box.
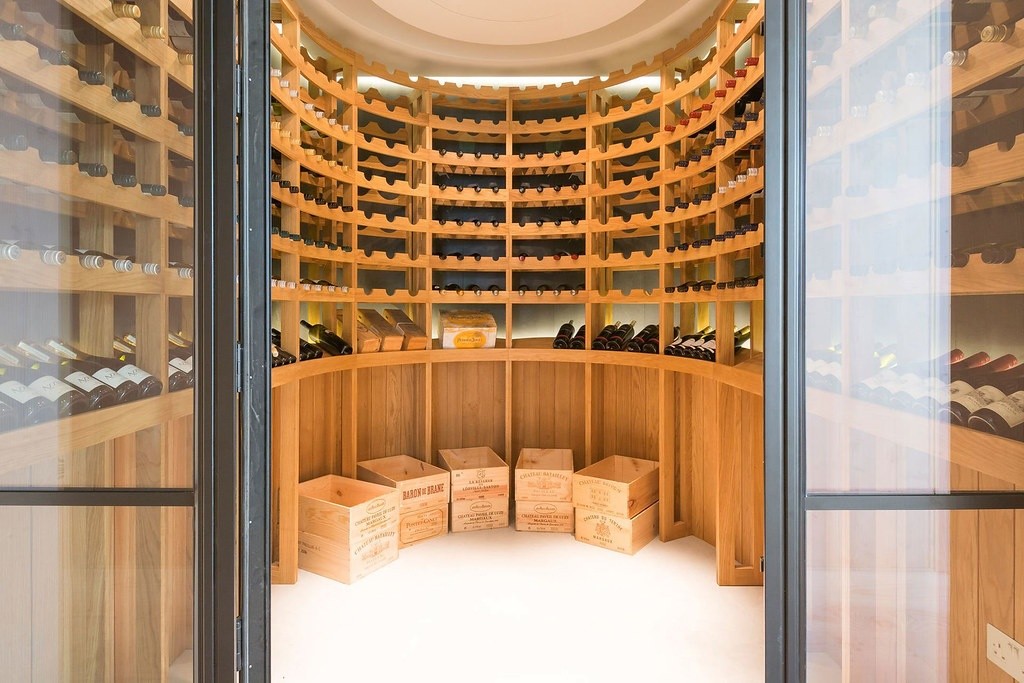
[437,310,497,349]
[335,308,427,353]
[297,449,658,585]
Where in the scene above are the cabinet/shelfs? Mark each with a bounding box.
[2,1,1024,683]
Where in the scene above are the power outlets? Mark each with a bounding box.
[987,624,1024,683]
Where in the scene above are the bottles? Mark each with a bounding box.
[0,0,200,432]
[552,320,750,361]
[790,0,1023,442]
[663,55,765,294]
[433,149,583,296]
[267,66,352,366]
